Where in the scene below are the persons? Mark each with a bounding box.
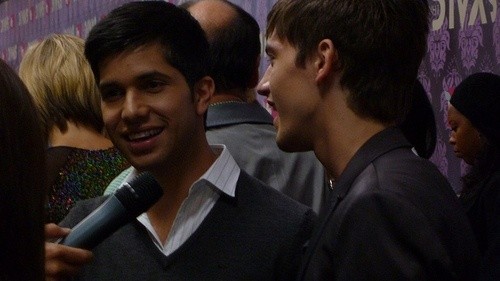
[258,0,480,281]
[19,34,131,224]
[447,72,500,259]
[178,0,334,215]
[0,58,92,281]
[56,0,318,281]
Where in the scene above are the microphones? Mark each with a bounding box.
[58,171,163,251]
